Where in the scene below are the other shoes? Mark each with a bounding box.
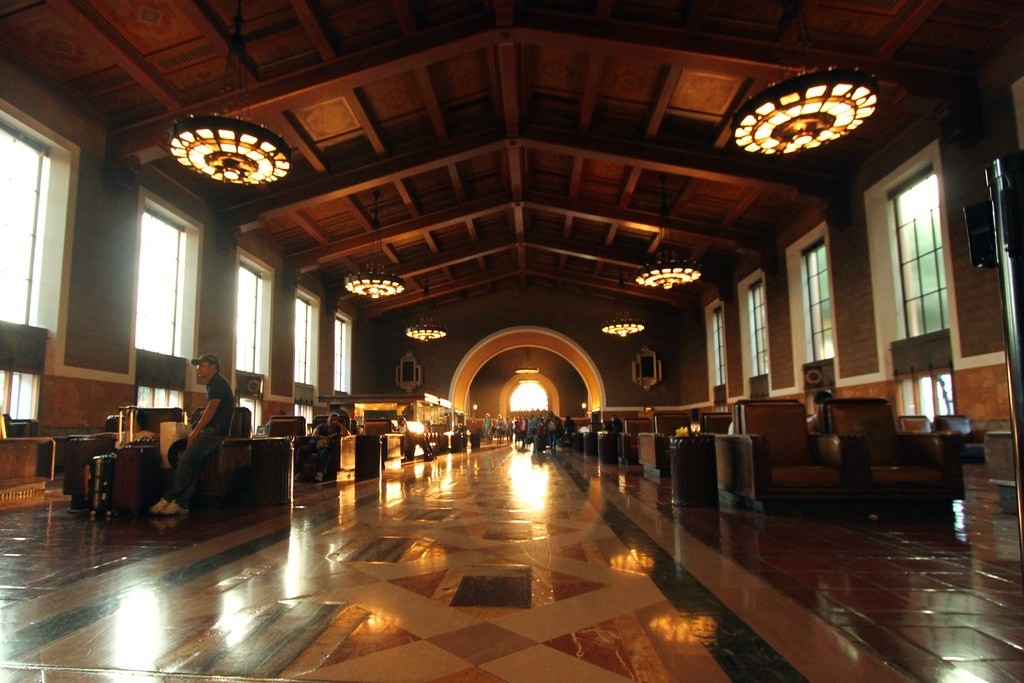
[294,473,302,482]
[315,472,323,481]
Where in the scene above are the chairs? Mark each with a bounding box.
[59,407,403,511]
[618,396,987,518]
[0,413,55,496]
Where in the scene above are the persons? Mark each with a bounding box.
[806,390,833,433]
[150,353,235,514]
[398,417,436,462]
[294,414,351,481]
[610,415,623,432]
[482,412,575,455]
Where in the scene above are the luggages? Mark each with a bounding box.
[534,427,545,450]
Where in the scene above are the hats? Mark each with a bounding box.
[191,354,220,372]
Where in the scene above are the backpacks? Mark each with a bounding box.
[548,419,556,431]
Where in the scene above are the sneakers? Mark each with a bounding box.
[150,498,169,513]
[160,500,189,515]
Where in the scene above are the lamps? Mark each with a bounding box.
[600,273,646,337]
[343,190,407,300]
[166,1,294,186]
[632,174,704,292]
[406,278,448,341]
[732,1,879,156]
